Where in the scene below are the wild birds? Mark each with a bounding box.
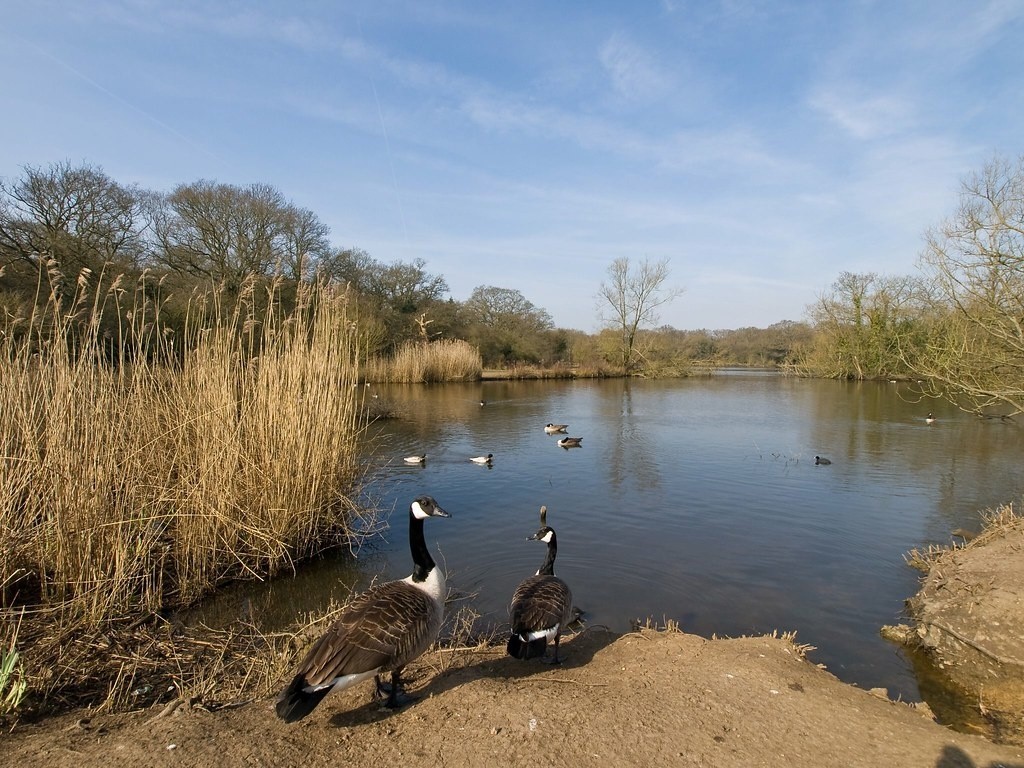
[479,400,486,409]
[403,453,427,463]
[557,437,584,446]
[544,423,569,434]
[469,453,493,463]
[506,526,572,664]
[926,412,935,425]
[275,493,452,725]
[352,381,378,399]
[815,455,832,465]
